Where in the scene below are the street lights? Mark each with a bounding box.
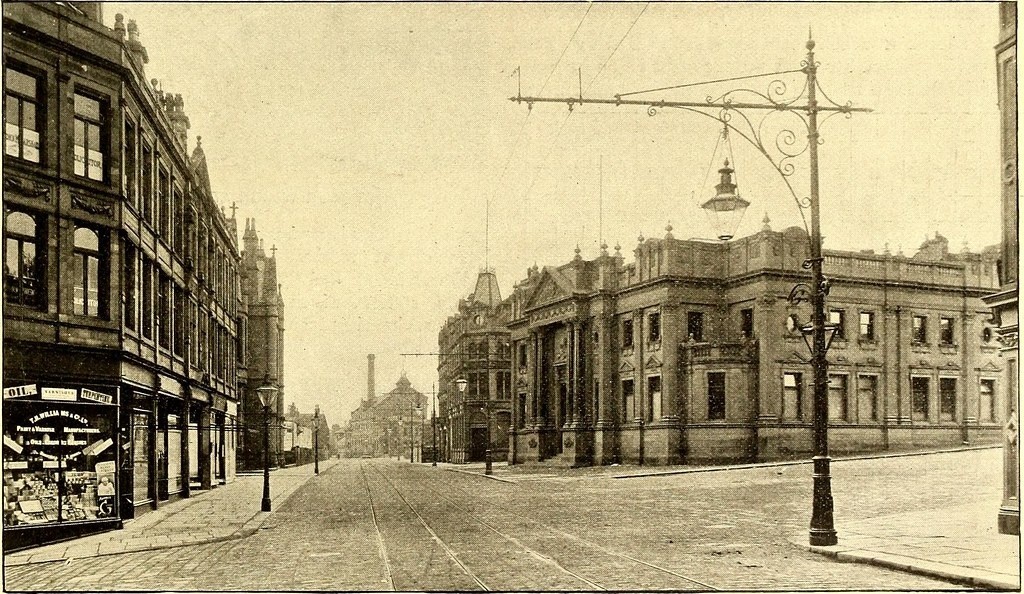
[401,332,508,477]
[386,384,437,469]
[311,412,320,475]
[510,24,876,545]
[252,373,278,512]
